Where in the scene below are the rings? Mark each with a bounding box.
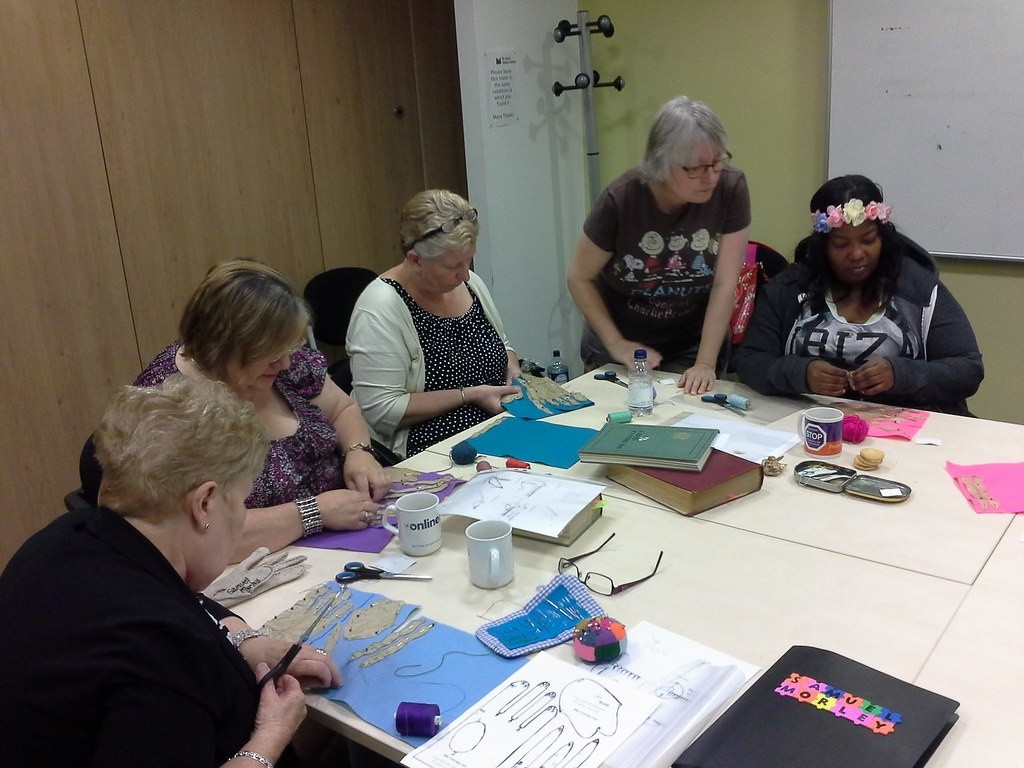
[316,648,328,656]
[366,511,368,521]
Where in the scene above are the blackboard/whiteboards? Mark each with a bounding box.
[826,0,1024,263]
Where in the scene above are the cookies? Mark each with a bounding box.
[853,448,885,471]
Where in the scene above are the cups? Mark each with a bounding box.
[797,407,844,459]
[382,491,441,556]
[465,519,514,590]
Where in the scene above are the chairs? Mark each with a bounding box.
[304,267,380,396]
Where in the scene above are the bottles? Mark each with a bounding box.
[628,349,654,417]
[547,350,569,383]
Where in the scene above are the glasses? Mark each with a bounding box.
[556,532,664,597]
[673,150,732,180]
[409,208,478,251]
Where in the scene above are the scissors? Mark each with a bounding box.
[593,371,628,388]
[335,561,434,584]
[256,597,334,691]
[701,393,746,416]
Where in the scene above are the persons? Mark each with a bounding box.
[345,189,523,459]
[0,374,343,768]
[130,254,392,567]
[733,175,985,419]
[566,94,751,395]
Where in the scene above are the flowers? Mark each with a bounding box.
[810,198,893,233]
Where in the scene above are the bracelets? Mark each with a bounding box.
[228,750,274,768]
[232,628,270,651]
[343,443,374,456]
[292,493,324,538]
[460,388,466,405]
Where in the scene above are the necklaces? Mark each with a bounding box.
[191,357,213,381]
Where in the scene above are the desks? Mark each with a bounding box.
[201,362,1024,768]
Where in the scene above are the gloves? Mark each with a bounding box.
[202,546,308,607]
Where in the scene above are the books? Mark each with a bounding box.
[605,449,764,517]
[578,421,720,471]
[512,493,603,547]
[670,646,960,768]
